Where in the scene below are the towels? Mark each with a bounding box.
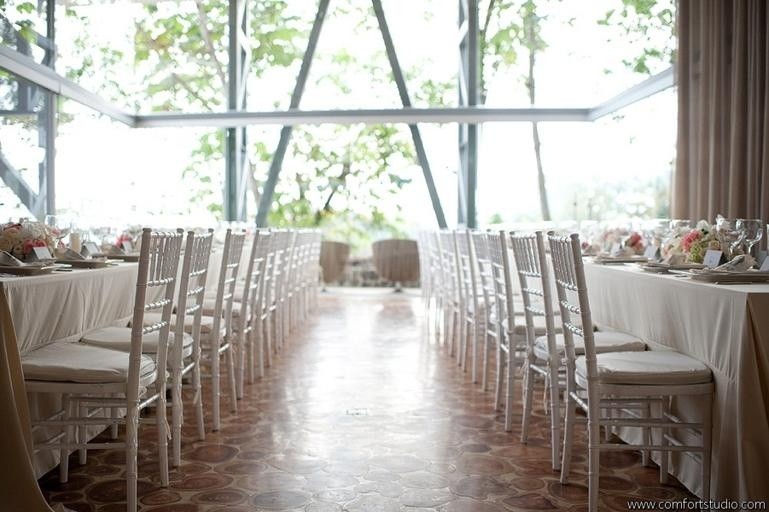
[1,250,54,266]
[64,245,92,261]
[111,244,125,255]
[705,254,757,273]
[661,253,688,265]
[622,247,635,257]
[584,244,592,254]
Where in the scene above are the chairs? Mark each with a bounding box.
[174,227,272,400]
[22,226,184,512]
[486,228,597,433]
[271,225,323,355]
[126,228,246,432]
[454,229,495,372]
[78,227,213,469]
[509,231,651,472]
[466,228,559,392]
[547,230,714,510]
[416,230,455,356]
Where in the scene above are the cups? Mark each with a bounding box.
[669,218,692,230]
[45,214,72,247]
[716,217,744,260]
[744,219,764,254]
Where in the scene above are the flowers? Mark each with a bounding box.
[664,226,720,264]
[1,219,61,256]
[117,225,156,251]
[613,233,648,257]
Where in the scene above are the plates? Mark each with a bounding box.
[668,263,709,270]
[57,258,126,263]
[0,263,73,271]
